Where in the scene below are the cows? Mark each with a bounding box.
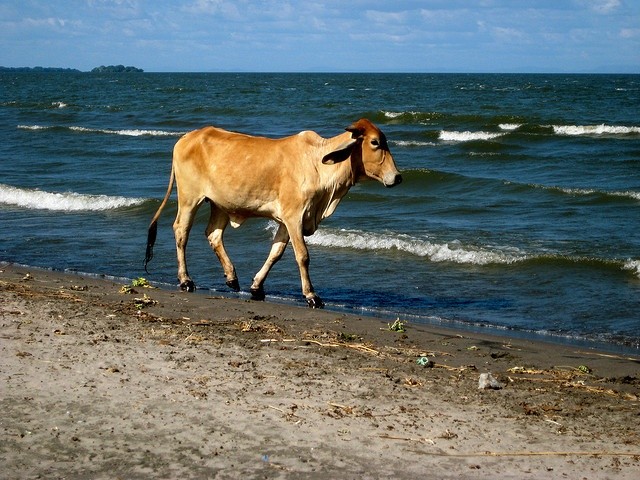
[145,118,403,308]
